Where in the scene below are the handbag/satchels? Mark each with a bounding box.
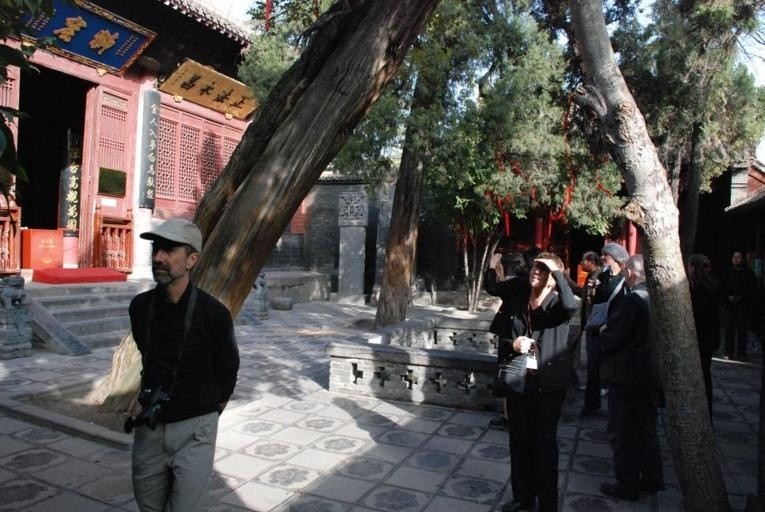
[585,303,608,328]
[493,350,526,396]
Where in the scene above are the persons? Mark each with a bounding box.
[720,249,756,362]
[598,253,667,502]
[127,216,241,512]
[482,245,543,434]
[563,249,602,416]
[687,252,722,429]
[584,239,630,422]
[498,249,580,512]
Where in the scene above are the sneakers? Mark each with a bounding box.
[600,475,661,501]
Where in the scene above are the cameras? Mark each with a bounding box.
[124,383,176,433]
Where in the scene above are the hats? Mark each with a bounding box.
[139,217,203,254]
[601,243,630,263]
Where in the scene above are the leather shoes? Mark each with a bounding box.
[502,499,558,512]
